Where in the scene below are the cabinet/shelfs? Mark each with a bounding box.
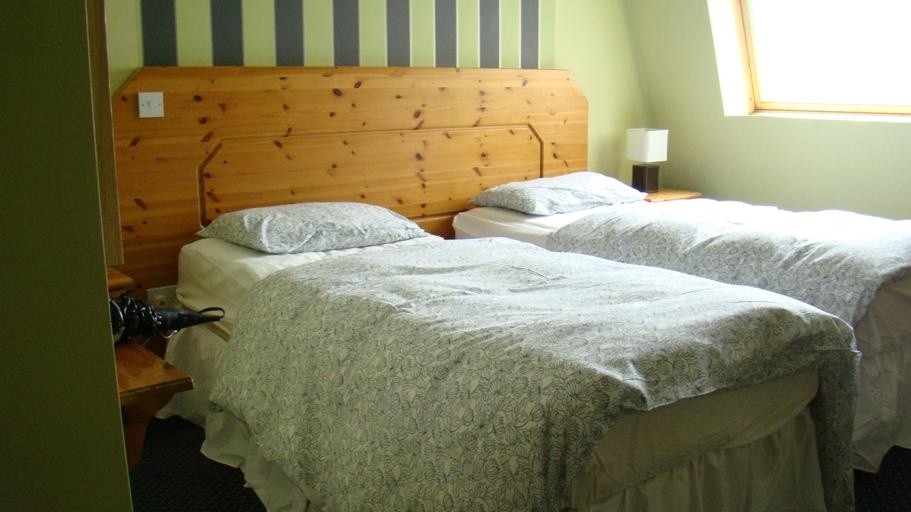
[0,0,194,512]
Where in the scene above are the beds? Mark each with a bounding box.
[453,200,911,476]
[154,238,862,512]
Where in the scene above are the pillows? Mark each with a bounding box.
[470,171,648,217]
[197,202,429,253]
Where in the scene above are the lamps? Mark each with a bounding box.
[627,126,668,192]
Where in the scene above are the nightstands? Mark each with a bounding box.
[645,187,704,204]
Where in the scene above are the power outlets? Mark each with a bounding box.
[147,285,179,312]
